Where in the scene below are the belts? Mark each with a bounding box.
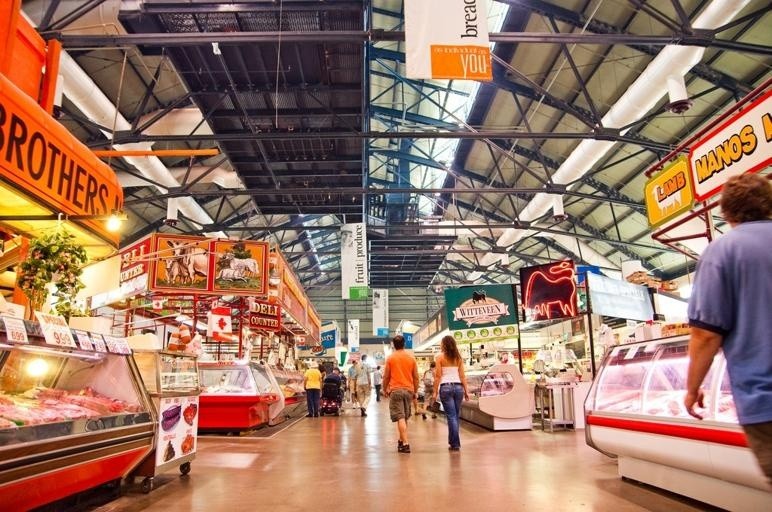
[440,383,462,386]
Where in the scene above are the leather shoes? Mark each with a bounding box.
[306,415,313,417]
[432,415,436,418]
[314,413,318,417]
[361,408,365,416]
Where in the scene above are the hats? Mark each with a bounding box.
[310,362,319,368]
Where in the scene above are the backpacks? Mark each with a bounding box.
[424,371,434,386]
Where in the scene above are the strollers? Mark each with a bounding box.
[319,373,344,417]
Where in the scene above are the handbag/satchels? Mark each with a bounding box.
[432,400,440,412]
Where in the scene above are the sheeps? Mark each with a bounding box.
[215,263,250,283]
[225,252,260,279]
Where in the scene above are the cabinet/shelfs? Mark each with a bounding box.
[198,359,304,437]
[583,333,771,511]
[0,316,201,512]
[416,334,595,434]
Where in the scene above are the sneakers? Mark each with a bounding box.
[352,404,356,409]
[398,440,403,452]
[402,444,410,453]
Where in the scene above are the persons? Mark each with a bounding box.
[410,395,420,416]
[373,364,384,402]
[303,362,321,417]
[684,172,772,490]
[422,362,438,419]
[432,335,470,451]
[320,364,326,379]
[381,335,420,453]
[354,354,372,416]
[347,359,358,404]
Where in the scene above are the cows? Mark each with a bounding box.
[167,239,209,286]
[472,290,487,305]
[160,256,188,287]
